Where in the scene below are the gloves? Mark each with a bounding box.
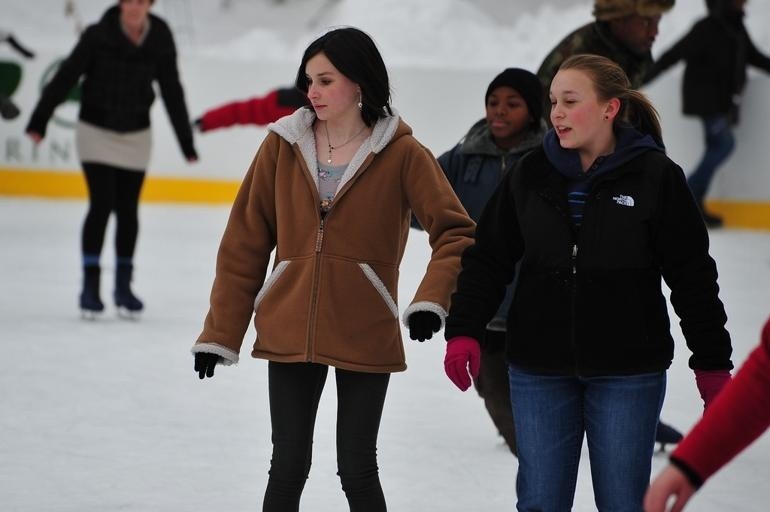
[446,338,480,392]
[408,311,441,342]
[193,353,218,378]
[694,370,730,410]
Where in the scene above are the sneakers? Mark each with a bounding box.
[80,291,104,311]
[655,421,684,445]
[114,286,144,311]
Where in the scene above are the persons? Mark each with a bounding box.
[637,0,770,230]
[188,26,477,512]
[655,417,682,444]
[408,65,555,462]
[191,78,304,134]
[638,316,769,511]
[442,51,733,511]
[23,0,197,312]
[526,0,676,109]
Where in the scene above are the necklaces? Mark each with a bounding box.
[322,117,370,163]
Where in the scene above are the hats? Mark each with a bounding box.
[484,67,545,120]
[593,1,675,23]
